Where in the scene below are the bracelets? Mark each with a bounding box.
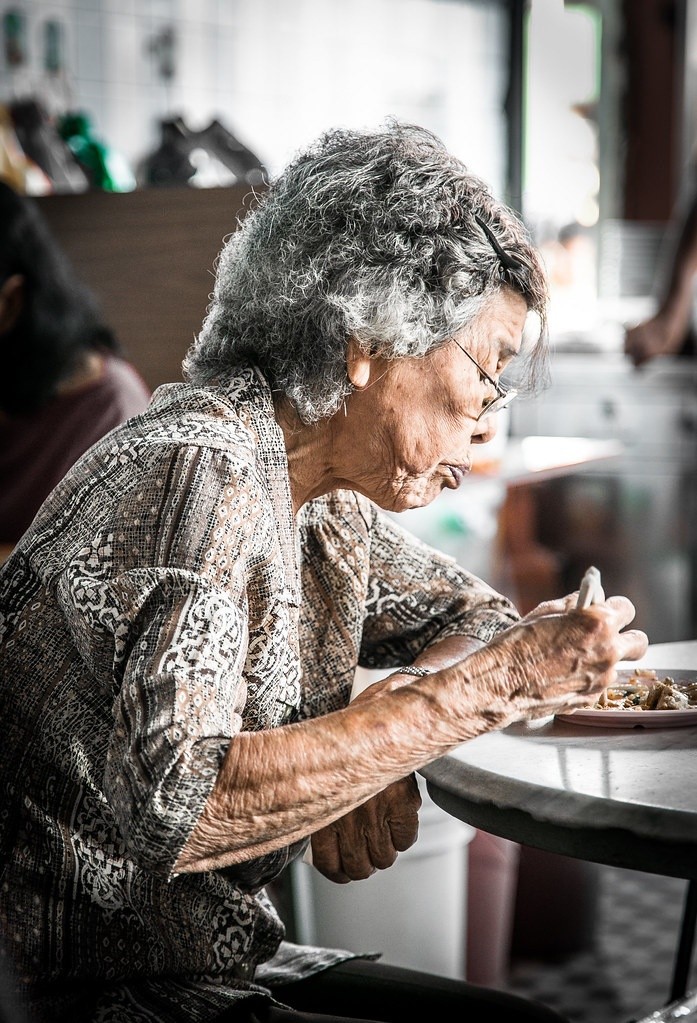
[390,665,440,676]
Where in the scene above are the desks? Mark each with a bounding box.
[416,639,697,1007]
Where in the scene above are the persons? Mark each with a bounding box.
[0,175,147,565]
[0,117,647,1023]
[624,192,697,372]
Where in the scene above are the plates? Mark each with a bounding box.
[550,668,697,728]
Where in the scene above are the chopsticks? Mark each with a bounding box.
[576,567,609,708]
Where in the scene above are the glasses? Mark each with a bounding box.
[447,334,517,421]
